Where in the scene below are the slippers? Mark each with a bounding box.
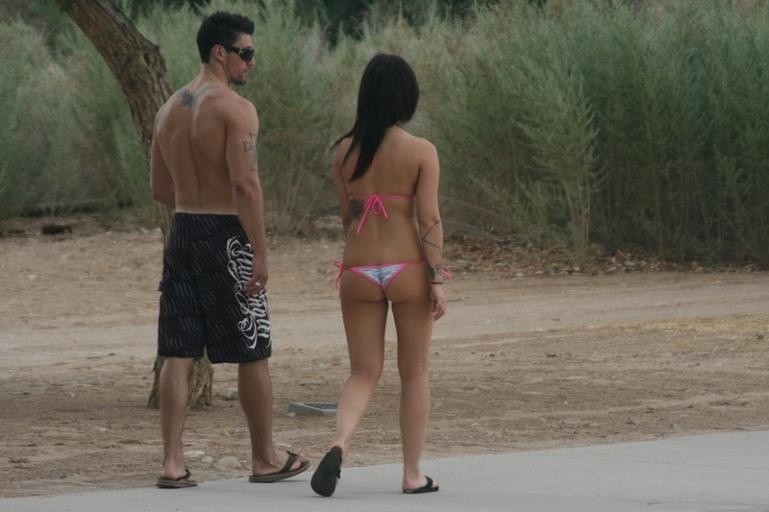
[249,451,310,483]
[403,476,438,493]
[311,451,342,496]
[156,469,198,487]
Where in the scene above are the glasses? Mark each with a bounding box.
[229,46,255,60]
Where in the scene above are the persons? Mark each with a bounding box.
[148,10,309,489]
[309,53,447,498]
